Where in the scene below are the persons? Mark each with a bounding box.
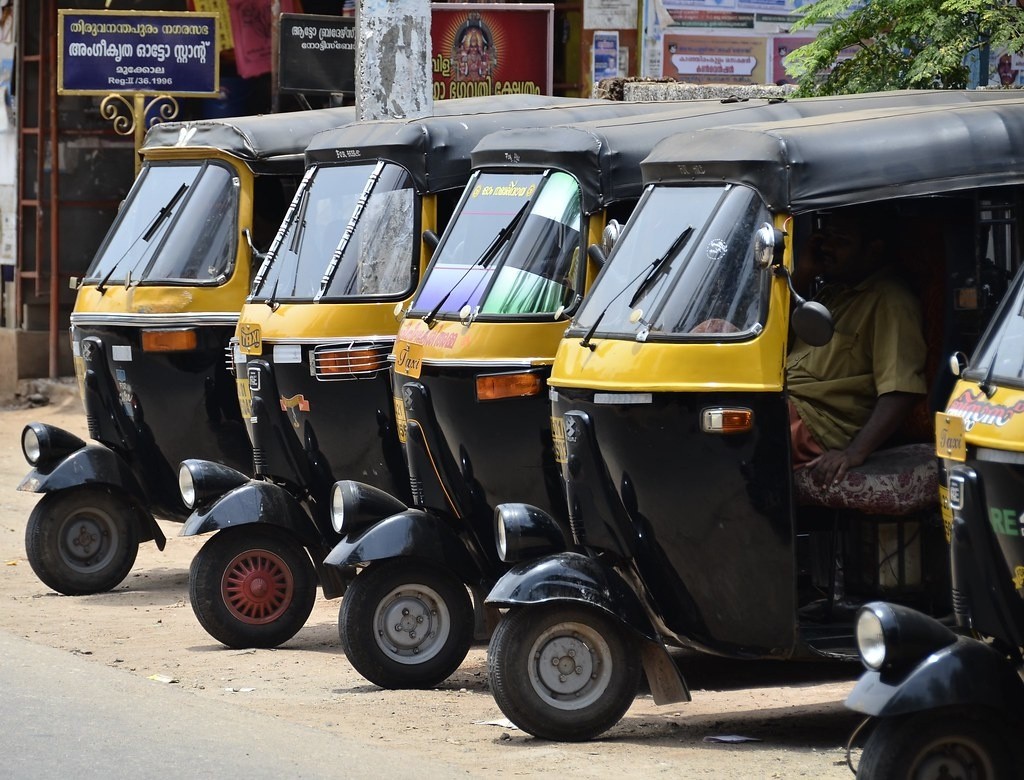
[694,208,928,491]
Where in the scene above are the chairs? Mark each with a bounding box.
[794,199,948,523]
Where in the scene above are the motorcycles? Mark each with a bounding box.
[844,252,1024,780]
[21,90,545,597]
[176,87,630,659]
[312,88,1024,693]
[486,98,1024,747]
